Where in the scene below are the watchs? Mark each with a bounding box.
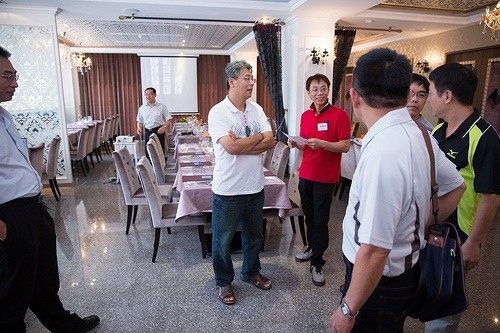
[341,298,359,320]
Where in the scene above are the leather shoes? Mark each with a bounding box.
[74,315,100,333]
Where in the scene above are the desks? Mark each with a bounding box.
[335,134,368,200]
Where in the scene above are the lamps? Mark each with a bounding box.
[310,40,328,66]
[478,1,500,43]
[58,32,73,64]
[415,54,431,74]
[73,40,92,75]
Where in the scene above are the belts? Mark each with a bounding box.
[145,124,162,131]
[0,193,43,210]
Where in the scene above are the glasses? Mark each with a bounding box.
[308,88,330,93]
[144,93,155,96]
[0,72,19,81]
[233,76,257,83]
[411,89,428,98]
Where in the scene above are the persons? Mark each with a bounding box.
[426,61,499,274]
[287,74,353,287]
[0,47,102,333]
[205,60,278,304]
[136,88,173,166]
[406,72,437,136]
[328,47,468,333]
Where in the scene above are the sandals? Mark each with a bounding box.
[217,283,236,305]
[240,271,273,289]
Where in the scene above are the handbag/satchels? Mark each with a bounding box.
[407,222,470,324]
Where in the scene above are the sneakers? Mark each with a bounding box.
[296,243,325,286]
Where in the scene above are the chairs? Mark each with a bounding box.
[19,109,309,264]
[350,121,359,138]
